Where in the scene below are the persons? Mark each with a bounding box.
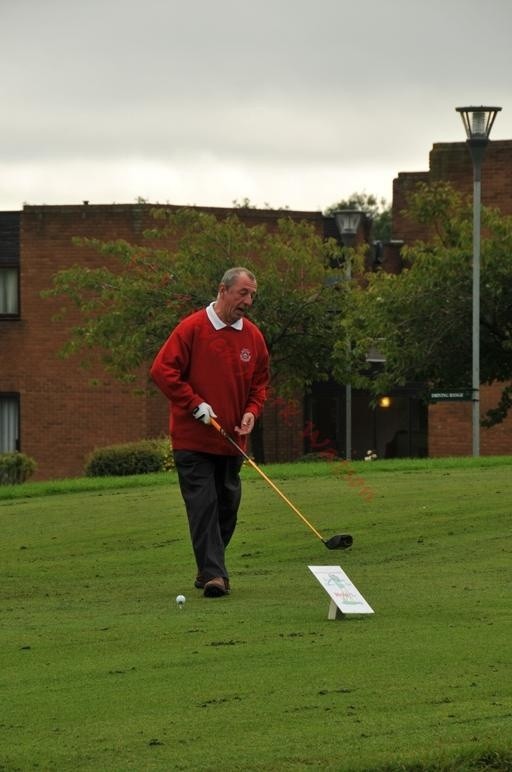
[149,266,271,597]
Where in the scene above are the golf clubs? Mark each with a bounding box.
[210,418,354,551]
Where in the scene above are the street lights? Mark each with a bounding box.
[332,208,366,461]
[455,105,503,464]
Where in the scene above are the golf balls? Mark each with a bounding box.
[175,595,186,604]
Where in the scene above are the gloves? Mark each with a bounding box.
[192,402,217,425]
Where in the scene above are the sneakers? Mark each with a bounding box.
[194,575,229,596]
